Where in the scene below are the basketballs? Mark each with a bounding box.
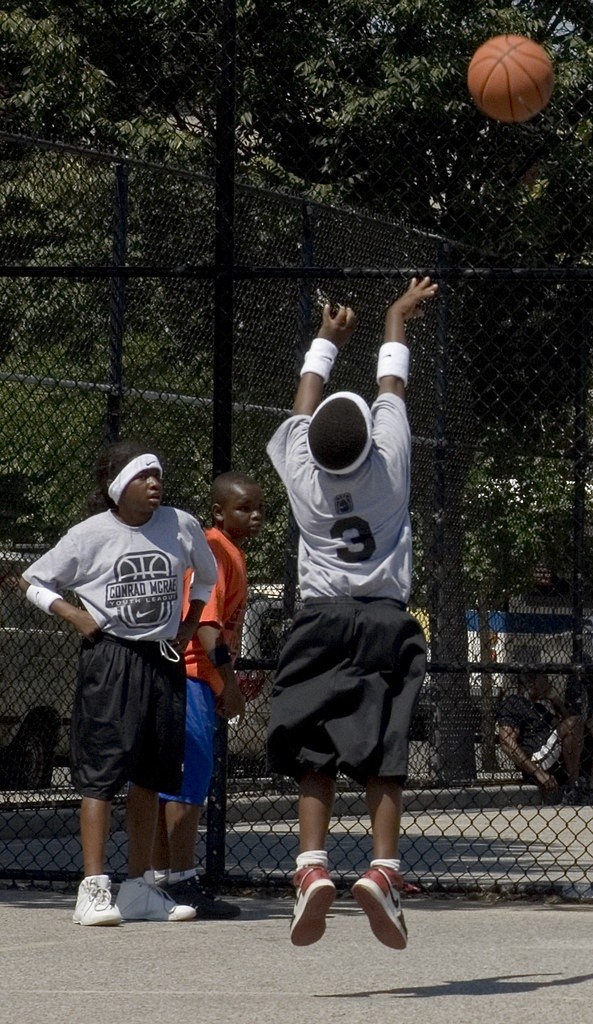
[466,31,555,125]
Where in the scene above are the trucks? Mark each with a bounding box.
[414,609,593,751]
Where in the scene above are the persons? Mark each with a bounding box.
[266,276,439,949]
[158,471,262,919]
[499,669,593,805]
[18,443,218,926]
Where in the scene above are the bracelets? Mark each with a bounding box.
[207,643,232,669]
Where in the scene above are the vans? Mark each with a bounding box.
[538,629,592,704]
[0,550,85,793]
[227,597,302,773]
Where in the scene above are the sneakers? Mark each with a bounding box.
[561,785,593,806]
[73,874,122,926]
[352,865,408,950]
[114,870,197,921]
[289,863,337,946]
[167,871,241,921]
